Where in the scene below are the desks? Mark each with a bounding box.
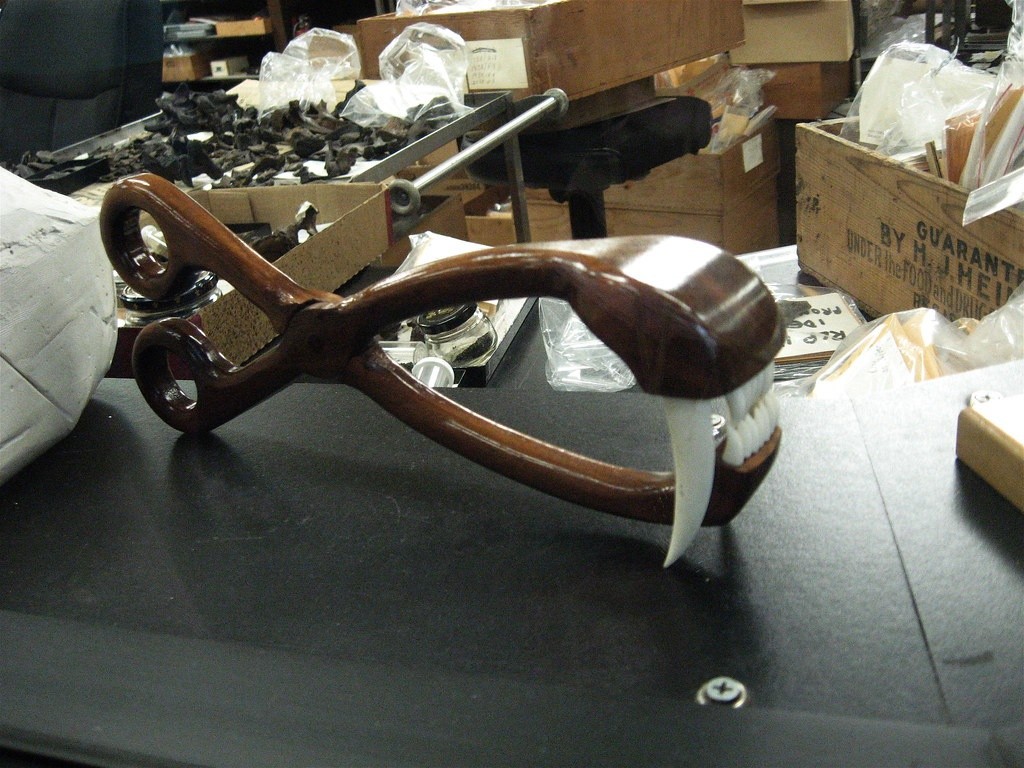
[0,376,1024,768]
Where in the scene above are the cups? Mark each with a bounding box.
[538,296,636,391]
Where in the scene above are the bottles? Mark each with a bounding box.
[417,301,498,367]
[119,270,222,326]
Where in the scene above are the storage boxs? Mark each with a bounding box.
[343,0,1023,329]
[66,180,393,377]
[162,16,272,82]
